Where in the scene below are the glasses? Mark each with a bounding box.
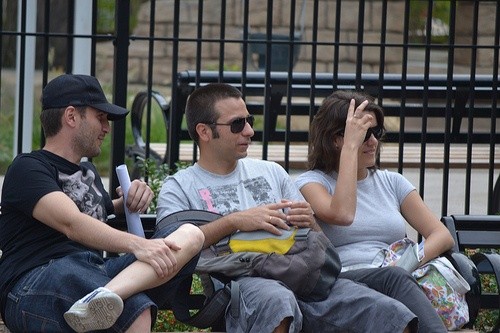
[201,115,255,133]
[337,125,384,144]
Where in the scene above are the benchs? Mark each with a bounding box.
[126,71,500,166]
[113,213,500,333]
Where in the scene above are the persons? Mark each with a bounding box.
[0,74,205,333]
[157,84,419,333]
[294,90,455,333]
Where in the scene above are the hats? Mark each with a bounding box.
[41,74,130,121]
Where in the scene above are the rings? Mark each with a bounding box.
[353,115,358,118]
[269,217,272,223]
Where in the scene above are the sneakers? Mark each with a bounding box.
[64,286,124,333]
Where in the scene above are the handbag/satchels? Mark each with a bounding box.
[150,210,341,329]
[371,237,470,333]
